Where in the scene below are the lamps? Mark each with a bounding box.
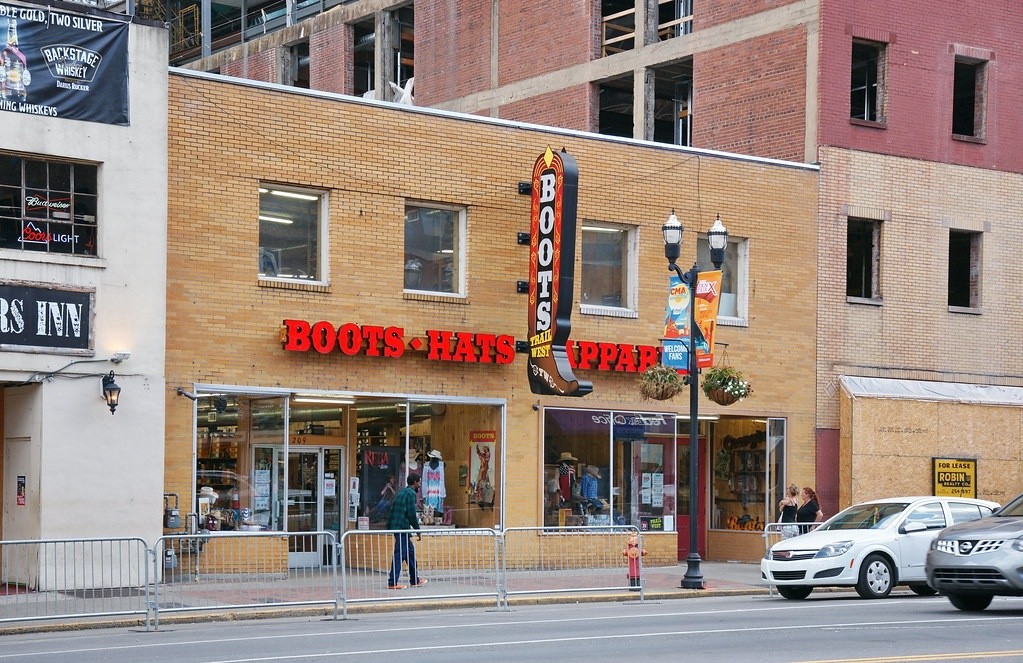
[113,351,132,363]
[100,371,122,415]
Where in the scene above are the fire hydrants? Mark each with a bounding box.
[620,530,648,590]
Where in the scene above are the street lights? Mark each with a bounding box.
[660,205,728,590]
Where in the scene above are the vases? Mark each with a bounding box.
[708,388,740,406]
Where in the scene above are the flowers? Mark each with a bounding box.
[700,365,754,403]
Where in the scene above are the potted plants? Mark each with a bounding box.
[634,343,682,401]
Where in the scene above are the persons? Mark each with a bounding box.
[399,449,447,518]
[387,473,428,589]
[555,452,601,515]
[364,474,397,522]
[547,475,557,501]
[779,484,823,541]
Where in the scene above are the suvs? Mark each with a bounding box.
[196,469,263,517]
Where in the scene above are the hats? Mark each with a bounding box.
[582,465,602,478]
[197,486,219,498]
[409,448,419,462]
[426,450,442,460]
[555,452,578,462]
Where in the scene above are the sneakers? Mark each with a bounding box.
[387,584,408,589]
[410,579,428,586]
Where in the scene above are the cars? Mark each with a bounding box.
[256,489,341,544]
[761,495,1004,600]
[925,492,1023,612]
